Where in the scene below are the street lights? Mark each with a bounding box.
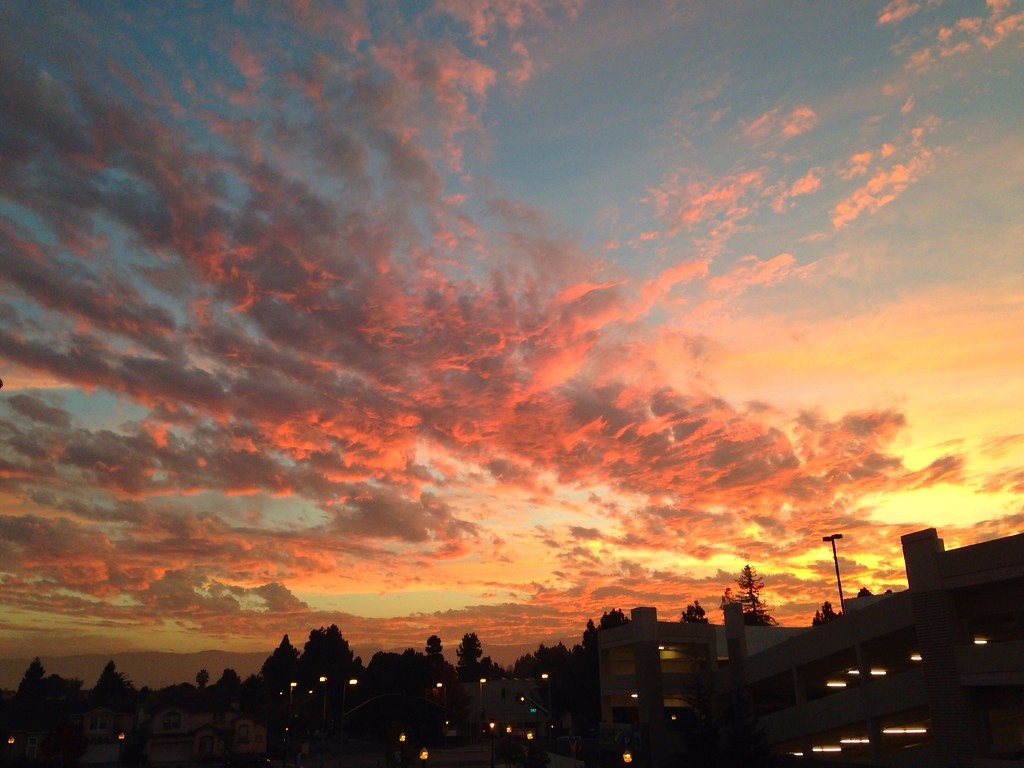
[288,682,297,729]
[116,731,125,768]
[319,676,328,768]
[541,672,553,749]
[478,678,488,749]
[7,735,16,763]
[822,533,845,614]
[398,733,408,768]
[622,748,635,768]
[418,747,430,768]
[338,679,358,768]
[436,682,447,748]
[488,720,498,768]
